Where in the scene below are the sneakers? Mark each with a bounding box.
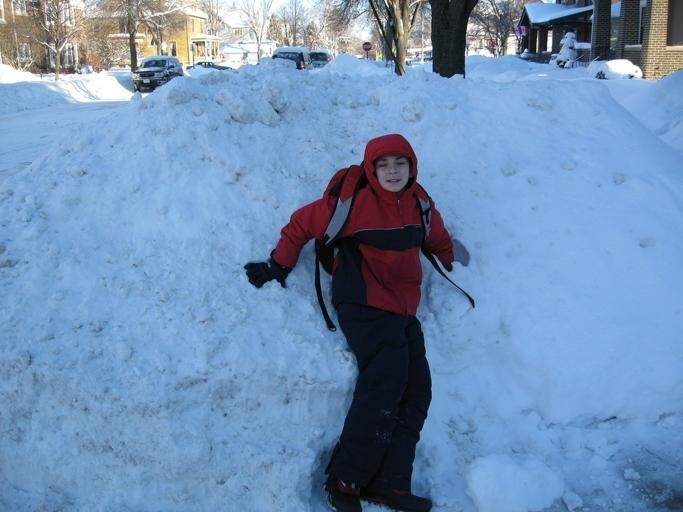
[322,473,362,512]
[362,475,432,511]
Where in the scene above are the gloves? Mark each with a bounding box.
[244,249,292,288]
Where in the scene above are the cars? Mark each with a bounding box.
[406,50,433,65]
[132,57,184,92]
[186,61,232,70]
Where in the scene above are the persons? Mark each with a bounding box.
[244,134,470,512]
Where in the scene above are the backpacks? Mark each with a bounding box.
[315,161,432,275]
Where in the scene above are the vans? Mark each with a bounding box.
[272,47,332,69]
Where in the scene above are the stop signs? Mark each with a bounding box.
[363,41,372,51]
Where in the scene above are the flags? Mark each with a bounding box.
[520,26,528,36]
[513,27,521,35]
